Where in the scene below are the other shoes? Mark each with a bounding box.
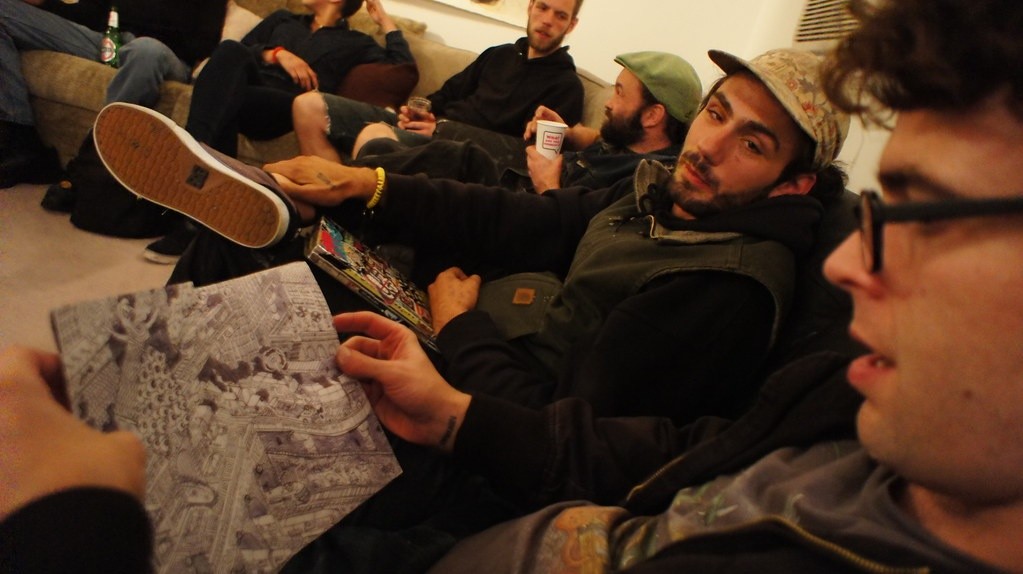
[0,145,65,189]
[41,158,92,212]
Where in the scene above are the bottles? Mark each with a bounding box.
[99,5,122,69]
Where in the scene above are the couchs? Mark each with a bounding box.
[17,0,872,364]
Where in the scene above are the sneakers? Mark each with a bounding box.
[143,222,197,263]
[93,101,302,250]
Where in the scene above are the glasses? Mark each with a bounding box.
[858,189,1023,275]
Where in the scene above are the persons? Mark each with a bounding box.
[0,0,1022,573]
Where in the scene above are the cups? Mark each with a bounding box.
[536,119,569,158]
[404,96,432,131]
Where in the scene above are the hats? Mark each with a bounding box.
[709,47,852,169]
[613,51,703,124]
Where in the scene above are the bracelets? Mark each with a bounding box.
[272,46,284,62]
[366,167,386,206]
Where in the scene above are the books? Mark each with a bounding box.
[314,216,439,345]
[306,239,442,354]
